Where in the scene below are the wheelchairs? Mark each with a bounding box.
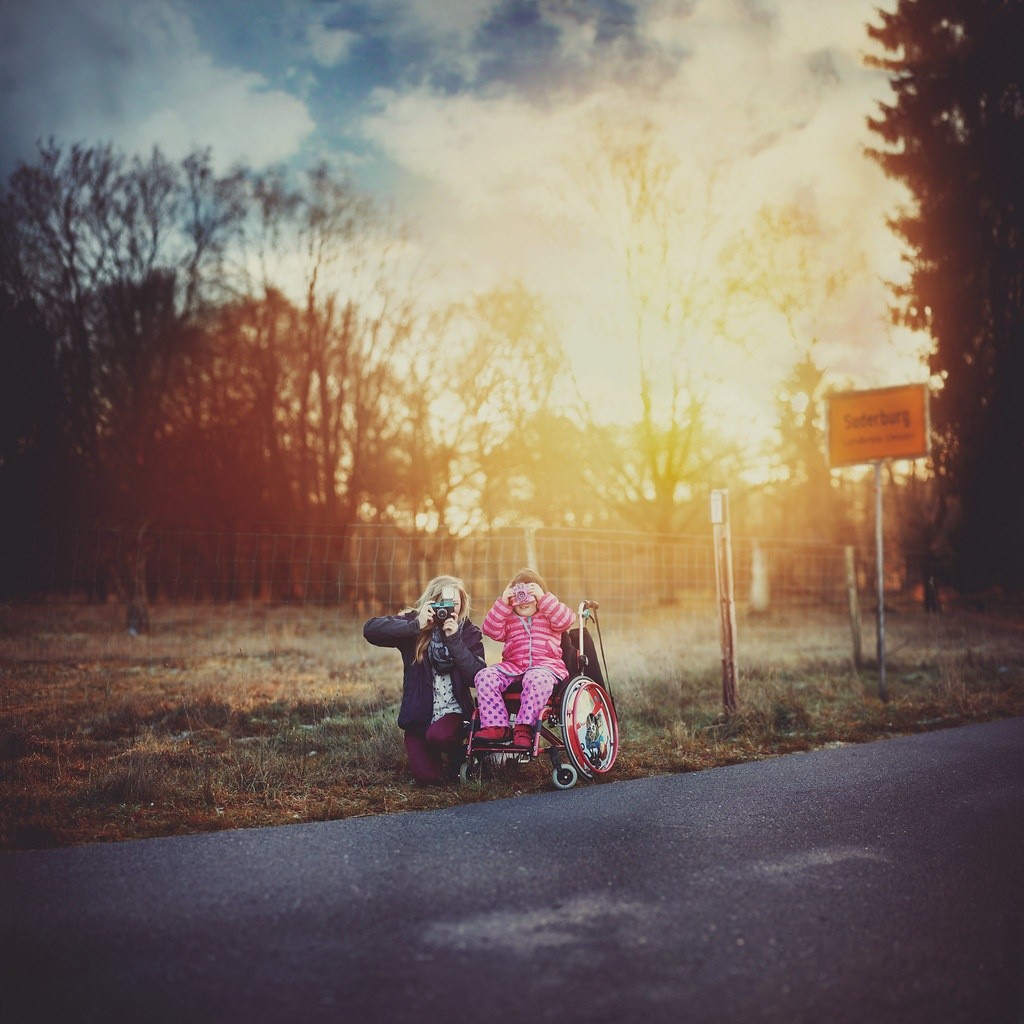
[458,598,620,792]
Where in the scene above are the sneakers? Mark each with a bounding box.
[512,724,532,748]
[473,727,513,742]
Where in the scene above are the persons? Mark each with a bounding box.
[920,536,949,617]
[475,569,576,747]
[363,574,486,787]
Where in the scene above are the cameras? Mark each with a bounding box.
[428,586,455,625]
[509,583,536,606]
